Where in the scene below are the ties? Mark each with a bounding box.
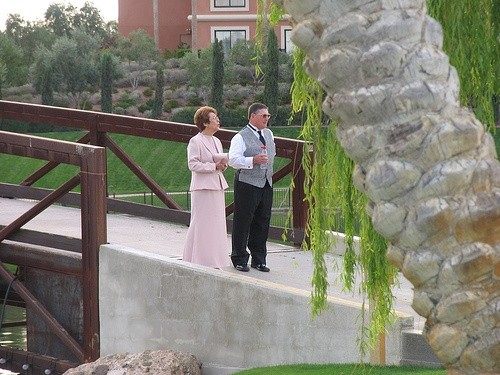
[248,124,266,147]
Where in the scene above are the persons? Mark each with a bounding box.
[228,103,276,272]
[182,106,231,272]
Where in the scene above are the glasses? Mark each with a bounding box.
[255,113,271,119]
[207,116,218,122]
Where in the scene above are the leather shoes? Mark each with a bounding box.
[251,263,270,272]
[235,264,250,272]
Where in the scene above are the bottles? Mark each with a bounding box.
[260,146,268,169]
